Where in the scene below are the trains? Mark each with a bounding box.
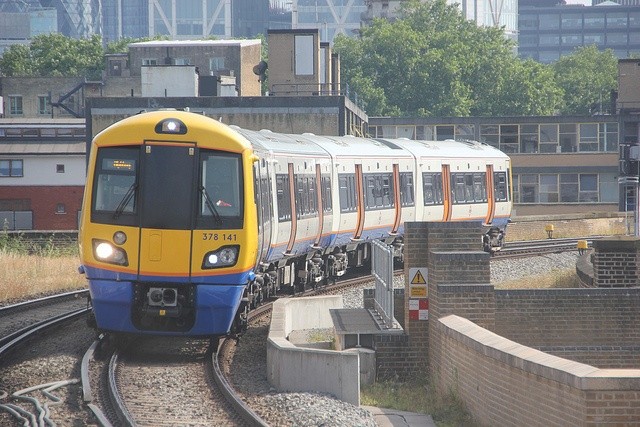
[78,106,514,351]
